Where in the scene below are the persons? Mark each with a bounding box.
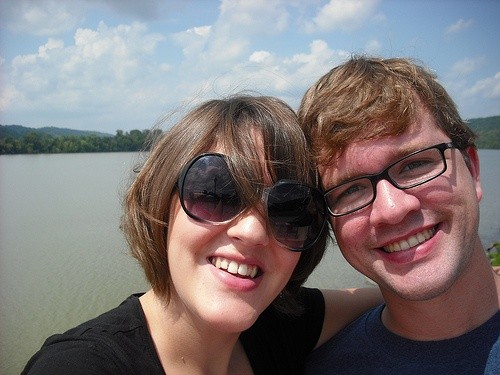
[296,56,500,375]
[19,95,500,375]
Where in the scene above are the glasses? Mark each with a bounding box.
[321,141,461,217]
[174,152,328,252]
